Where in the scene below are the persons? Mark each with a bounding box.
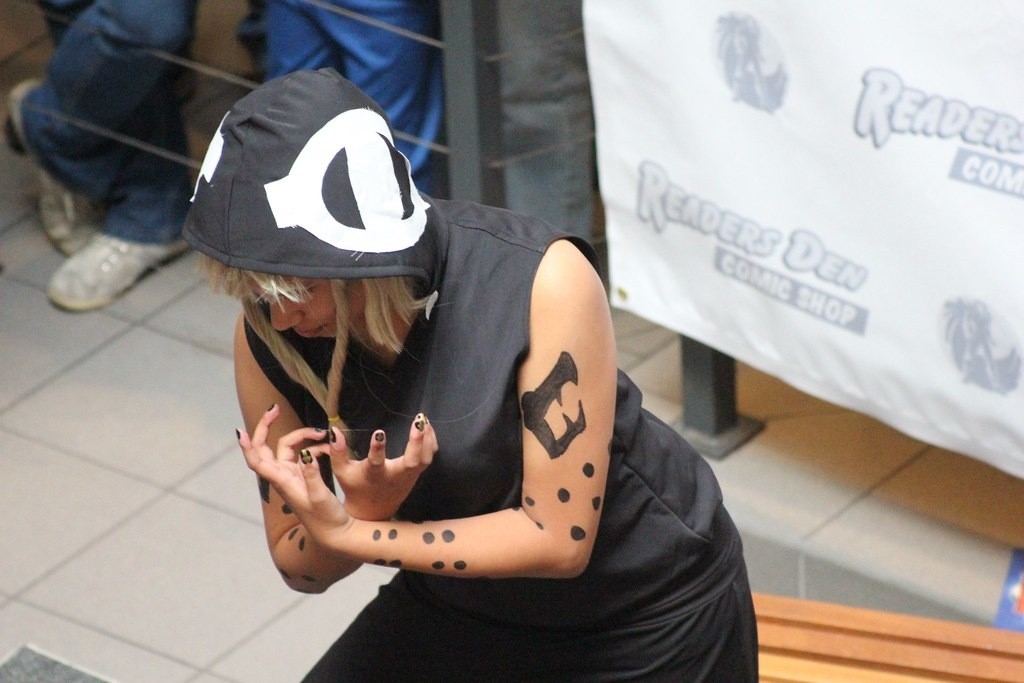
[470,1,593,241]
[234,0,445,195]
[6,1,195,314]
[182,66,760,682]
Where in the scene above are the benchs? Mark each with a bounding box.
[751,591,1024,683]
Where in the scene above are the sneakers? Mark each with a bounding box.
[46,232,189,310]
[9,80,99,256]
